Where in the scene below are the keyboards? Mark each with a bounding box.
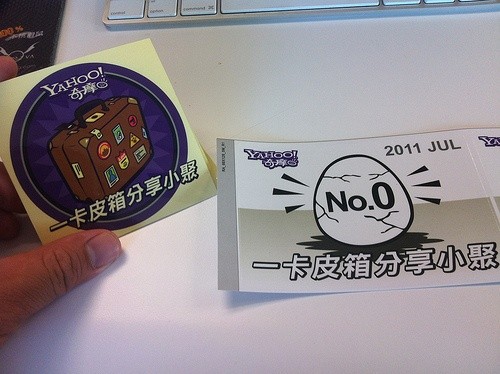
[100,0,499,31]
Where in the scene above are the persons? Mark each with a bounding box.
[0,52,120,347]
